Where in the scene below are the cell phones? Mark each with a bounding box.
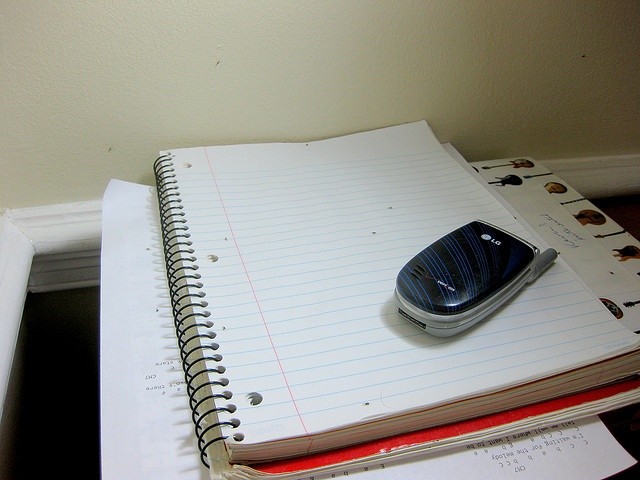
[392,219,558,337]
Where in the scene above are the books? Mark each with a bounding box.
[153,119,638,479]
